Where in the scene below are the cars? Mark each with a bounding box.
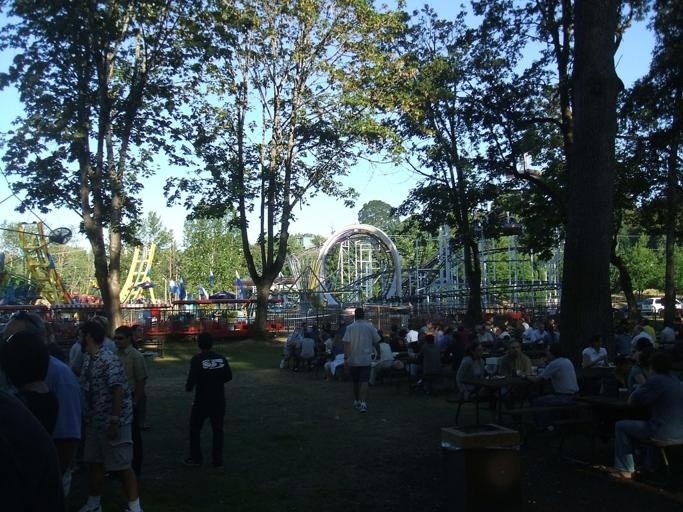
[638,297,682,315]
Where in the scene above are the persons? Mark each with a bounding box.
[90,316,117,351]
[407,333,427,360]
[625,339,657,396]
[3,310,83,495]
[77,320,142,512]
[406,326,419,345]
[417,320,431,341]
[1,389,65,512]
[605,352,683,481]
[582,333,609,388]
[132,324,145,357]
[368,336,394,386]
[629,316,683,348]
[455,342,503,420]
[416,334,443,396]
[280,324,347,383]
[341,307,380,413]
[184,333,232,467]
[521,344,581,435]
[436,311,560,349]
[389,324,403,352]
[500,338,532,402]
[3,332,60,435]
[113,325,146,475]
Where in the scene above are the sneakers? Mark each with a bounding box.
[353,400,361,410]
[122,506,143,512]
[184,458,201,466]
[103,472,113,480]
[78,504,102,512]
[214,465,224,473]
[359,401,368,413]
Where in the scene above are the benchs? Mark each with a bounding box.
[281,353,299,371]
[511,402,607,447]
[654,440,678,474]
[414,372,457,396]
[301,354,336,371]
[448,391,503,422]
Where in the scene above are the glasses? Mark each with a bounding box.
[113,336,128,340]
[7,335,15,345]
[8,311,39,329]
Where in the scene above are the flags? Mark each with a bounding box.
[235,270,246,299]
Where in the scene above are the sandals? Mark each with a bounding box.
[632,470,640,478]
[608,472,631,481]
